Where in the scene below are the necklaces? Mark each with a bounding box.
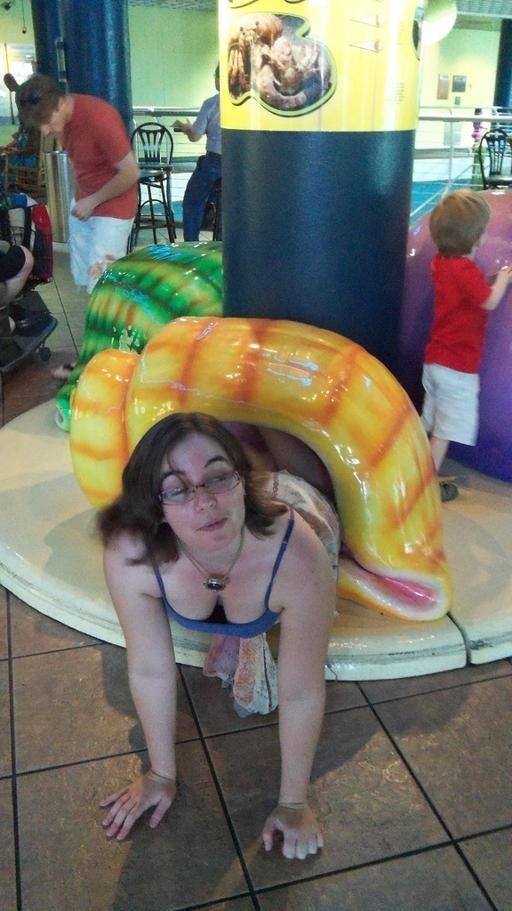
[168,526,245,594]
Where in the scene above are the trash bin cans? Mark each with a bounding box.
[45,150,73,243]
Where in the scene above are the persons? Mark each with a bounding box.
[172,65,220,243]
[415,187,512,505]
[11,73,144,386]
[92,411,346,864]
[1,72,54,312]
[468,108,489,143]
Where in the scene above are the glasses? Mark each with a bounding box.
[154,470,240,508]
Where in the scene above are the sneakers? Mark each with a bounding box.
[434,480,461,503]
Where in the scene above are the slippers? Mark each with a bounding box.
[47,359,78,383]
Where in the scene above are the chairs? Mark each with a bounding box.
[126,121,178,253]
[2,129,47,197]
[479,128,512,191]
